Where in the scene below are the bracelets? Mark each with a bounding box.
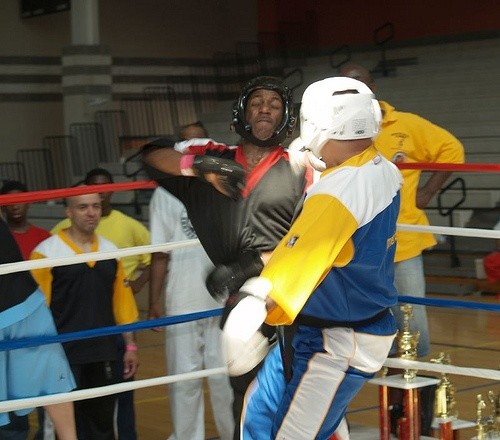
[126,344,137,351]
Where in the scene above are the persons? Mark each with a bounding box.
[0,180,52,440]
[140,76,299,440]
[148,124,233,440]
[49,168,152,440]
[220,77,403,440]
[339,64,464,437]
[29,185,139,440]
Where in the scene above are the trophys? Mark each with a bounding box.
[368,301,500,440]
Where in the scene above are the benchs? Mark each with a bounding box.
[26,37,500,228]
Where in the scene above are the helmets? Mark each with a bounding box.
[229,77,297,147]
[299,79,382,160]
